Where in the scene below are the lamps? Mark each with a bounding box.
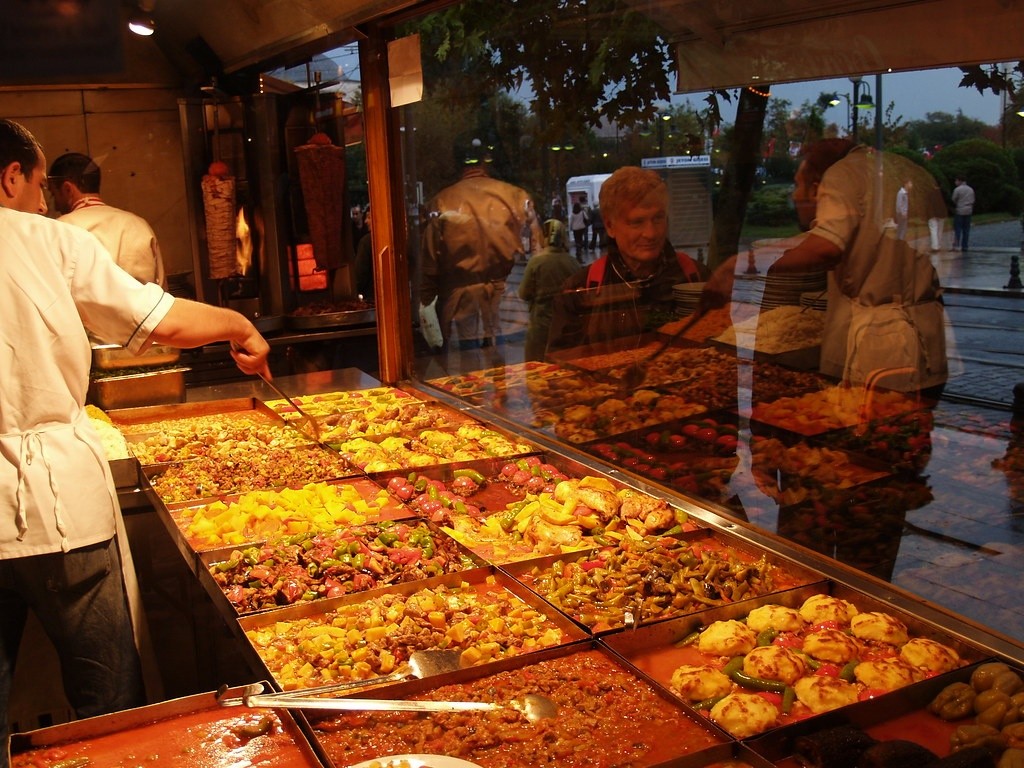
[128,0,154,36]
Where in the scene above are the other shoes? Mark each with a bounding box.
[962,246,968,251]
[953,241,960,247]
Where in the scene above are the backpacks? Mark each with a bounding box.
[820,234,930,466]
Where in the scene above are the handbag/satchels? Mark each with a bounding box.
[418,295,445,347]
[582,210,591,227]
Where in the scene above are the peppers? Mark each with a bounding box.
[214,521,475,604]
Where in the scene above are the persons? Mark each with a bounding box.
[46,151,165,351]
[697,115,964,572]
[418,132,546,380]
[949,173,977,251]
[341,166,739,504]
[749,139,912,584]
[0,118,272,719]
[923,180,950,252]
[895,179,912,241]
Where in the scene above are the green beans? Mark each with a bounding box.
[532,536,770,628]
[613,421,756,474]
[405,460,563,515]
[674,625,861,714]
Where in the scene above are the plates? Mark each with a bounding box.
[345,754,483,768]
[671,236,827,320]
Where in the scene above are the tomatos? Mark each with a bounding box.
[385,474,482,523]
[588,432,689,480]
[681,416,768,450]
[502,455,570,495]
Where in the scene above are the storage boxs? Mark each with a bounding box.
[95,346,181,367]
[92,366,192,410]
